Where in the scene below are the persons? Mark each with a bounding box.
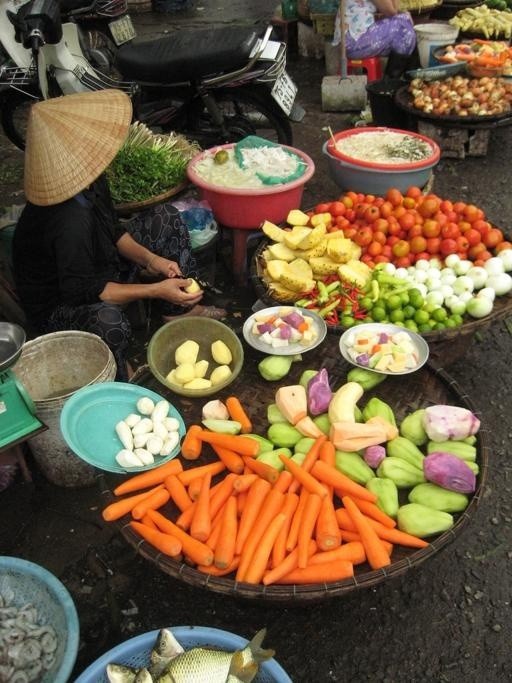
[7,87,229,362]
[331,0,418,79]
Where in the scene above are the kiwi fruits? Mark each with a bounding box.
[213,150,229,164]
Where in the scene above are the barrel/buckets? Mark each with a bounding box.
[414,22,460,69]
[11,330,118,489]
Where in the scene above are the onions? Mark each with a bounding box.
[408,75,511,116]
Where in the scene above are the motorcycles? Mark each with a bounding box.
[1,1,305,151]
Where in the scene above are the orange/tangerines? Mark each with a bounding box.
[341,287,464,332]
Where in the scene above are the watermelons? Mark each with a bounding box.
[484,0,507,10]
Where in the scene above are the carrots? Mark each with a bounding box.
[101,397,429,587]
[438,38,511,66]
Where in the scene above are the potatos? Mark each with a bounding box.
[167,339,233,389]
[422,451,477,496]
[275,369,400,469]
[422,405,481,441]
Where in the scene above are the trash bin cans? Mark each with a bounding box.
[364,78,410,130]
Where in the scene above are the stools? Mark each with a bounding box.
[219,224,265,288]
[339,55,382,84]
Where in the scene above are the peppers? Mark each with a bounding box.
[295,266,411,327]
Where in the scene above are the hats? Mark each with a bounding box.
[21,85,136,206]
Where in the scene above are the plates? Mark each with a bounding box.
[339,322,429,375]
[62,382,186,475]
[243,307,327,358]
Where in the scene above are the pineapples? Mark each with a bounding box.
[257,209,373,303]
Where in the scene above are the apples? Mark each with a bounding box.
[286,187,512,268]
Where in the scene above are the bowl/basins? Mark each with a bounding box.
[322,128,442,195]
[186,143,315,230]
[147,317,244,397]
[0,321,27,370]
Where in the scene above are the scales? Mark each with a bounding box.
[0,321,50,454]
